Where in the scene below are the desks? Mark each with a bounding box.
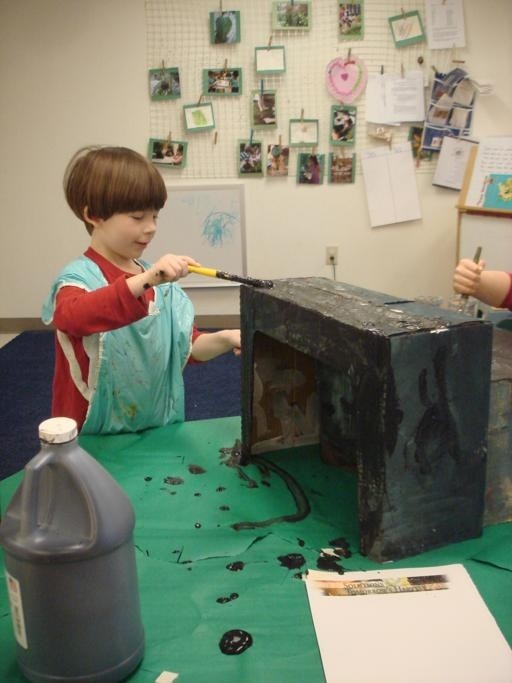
[0,380,511,682]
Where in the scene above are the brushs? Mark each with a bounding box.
[187,262,274,289]
[457,247,482,311]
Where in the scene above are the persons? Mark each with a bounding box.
[241,145,258,172]
[42,143,242,436]
[451,257,512,314]
[208,69,241,93]
[152,140,184,164]
[301,154,322,184]
[150,70,179,97]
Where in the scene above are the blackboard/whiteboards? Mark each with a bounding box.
[138,184,246,288]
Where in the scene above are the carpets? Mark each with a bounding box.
[0,326,244,481]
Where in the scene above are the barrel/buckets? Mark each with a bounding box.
[0,417,146,682]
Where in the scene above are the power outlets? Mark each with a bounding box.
[326,246,338,265]
